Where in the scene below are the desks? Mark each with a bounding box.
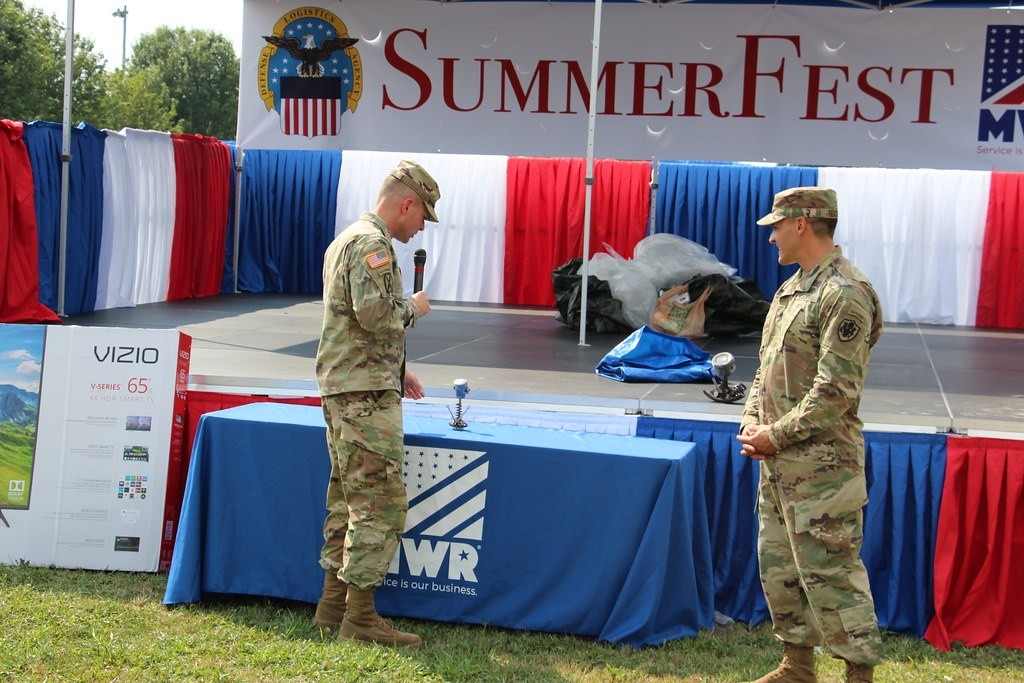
[162,401,715,650]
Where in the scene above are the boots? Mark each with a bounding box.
[313,572,348,629]
[750,641,816,683]
[841,658,874,683]
[338,587,422,648]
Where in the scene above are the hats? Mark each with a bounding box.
[756,187,838,226]
[390,159,440,223]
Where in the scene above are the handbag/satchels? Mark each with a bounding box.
[651,277,714,341]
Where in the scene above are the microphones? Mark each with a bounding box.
[414,249,426,294]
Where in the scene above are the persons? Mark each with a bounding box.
[312,160,440,647]
[736,186,886,683]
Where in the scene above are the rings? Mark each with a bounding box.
[406,389,410,391]
